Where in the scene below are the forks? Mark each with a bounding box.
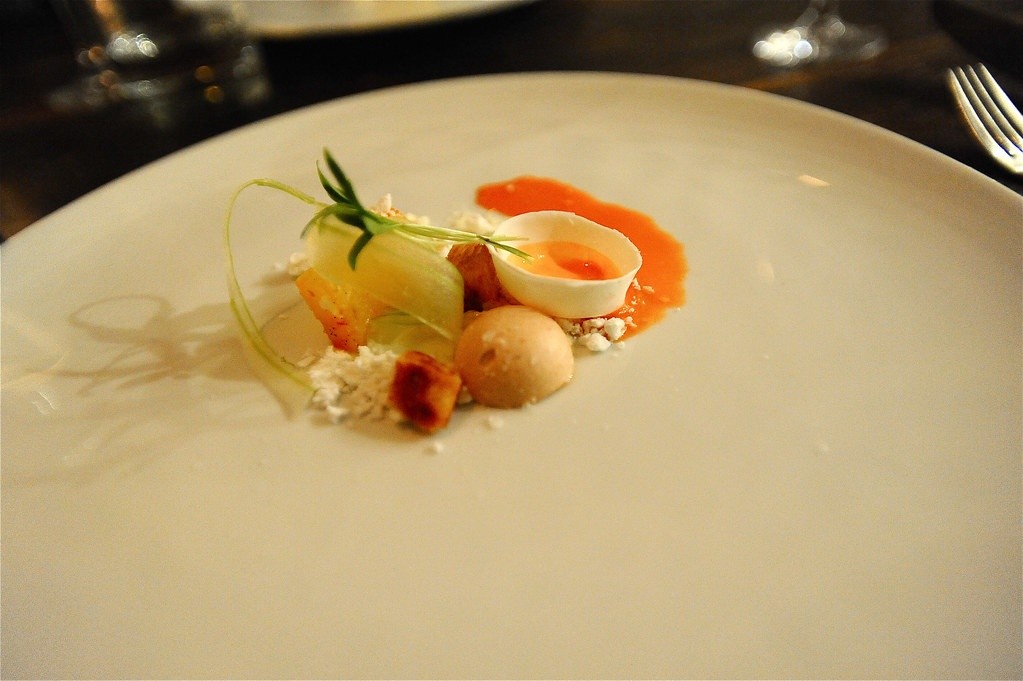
[945,61,1023,174]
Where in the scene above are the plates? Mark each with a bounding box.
[95,0,516,36]
[0,72,1023,681]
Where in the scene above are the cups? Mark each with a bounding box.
[105,0,276,154]
